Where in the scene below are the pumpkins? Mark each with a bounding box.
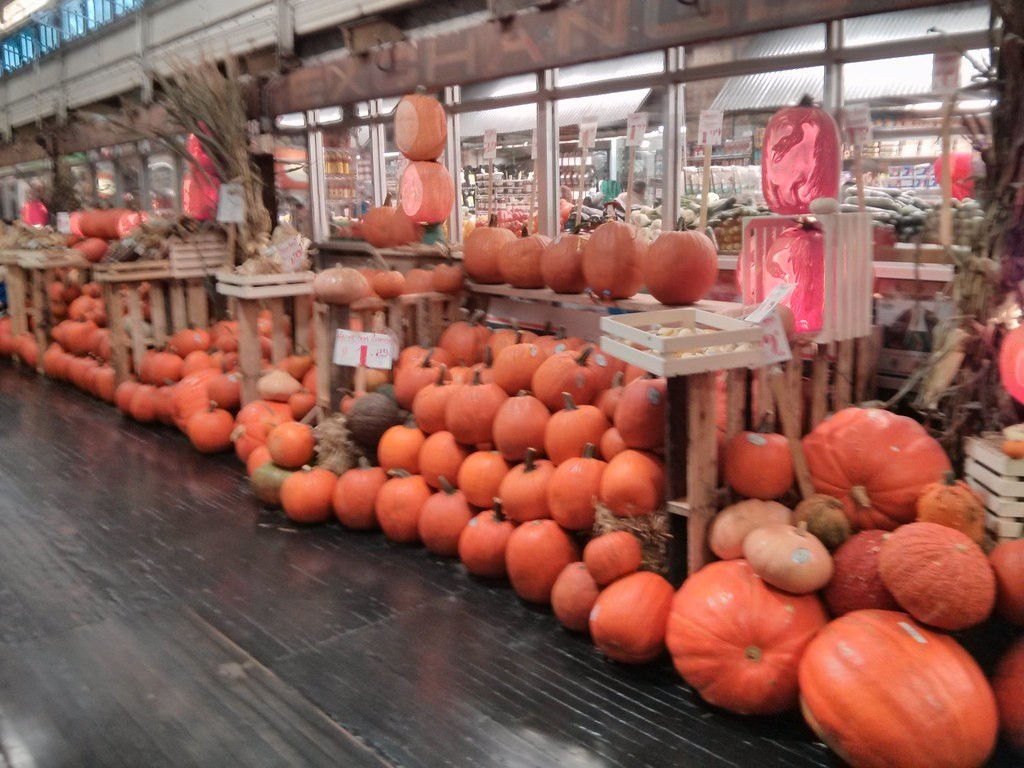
[0,193,1020,768]
